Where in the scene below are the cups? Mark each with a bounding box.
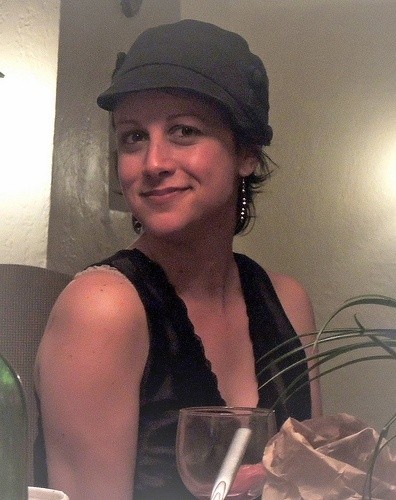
[175,407,278,500]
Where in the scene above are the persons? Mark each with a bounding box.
[30,19,320,500]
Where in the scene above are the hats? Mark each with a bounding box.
[96,19,274,147]
